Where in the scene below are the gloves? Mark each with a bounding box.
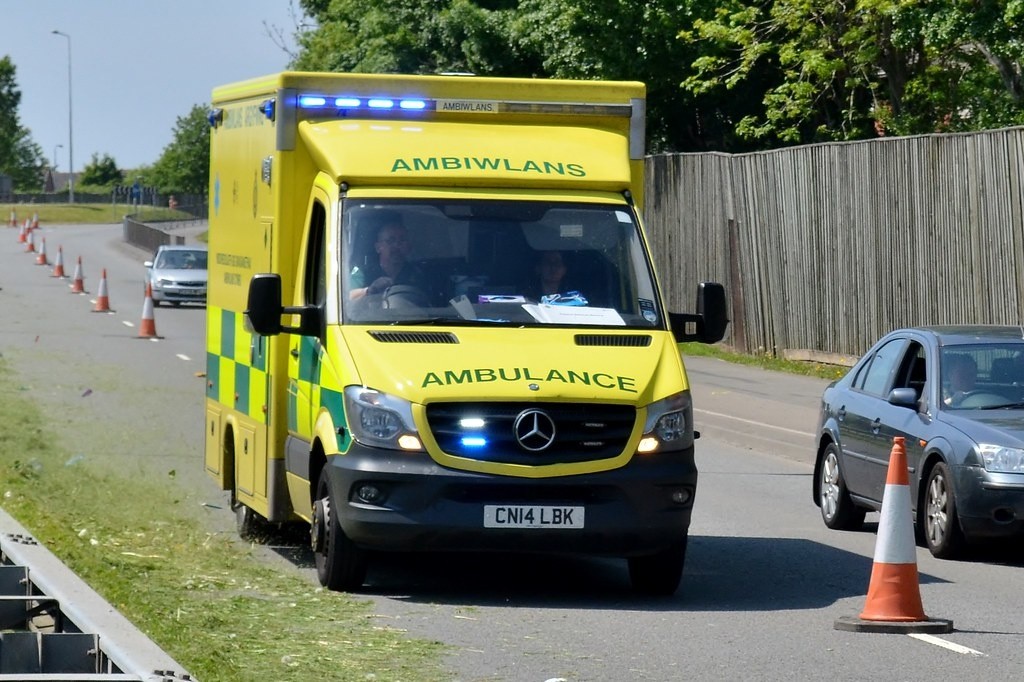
[366,277,393,294]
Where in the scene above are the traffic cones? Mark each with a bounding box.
[69,256,89,293]
[833,438,954,633]
[9,207,17,228]
[91,268,118,313]
[31,210,41,229]
[132,283,165,338]
[34,237,51,264]
[17,220,26,242]
[25,229,39,253]
[26,214,33,233]
[49,245,70,278]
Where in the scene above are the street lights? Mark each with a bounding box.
[54,145,64,200]
[53,30,75,204]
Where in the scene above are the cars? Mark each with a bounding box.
[144,245,209,306]
[813,325,1024,556]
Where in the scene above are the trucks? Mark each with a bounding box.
[202,71,730,596]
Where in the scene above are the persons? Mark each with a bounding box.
[940,357,985,404]
[347,219,428,308]
[169,195,177,209]
[520,246,580,302]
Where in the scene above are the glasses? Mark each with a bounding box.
[379,237,412,245]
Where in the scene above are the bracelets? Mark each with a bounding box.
[365,287,370,295]
